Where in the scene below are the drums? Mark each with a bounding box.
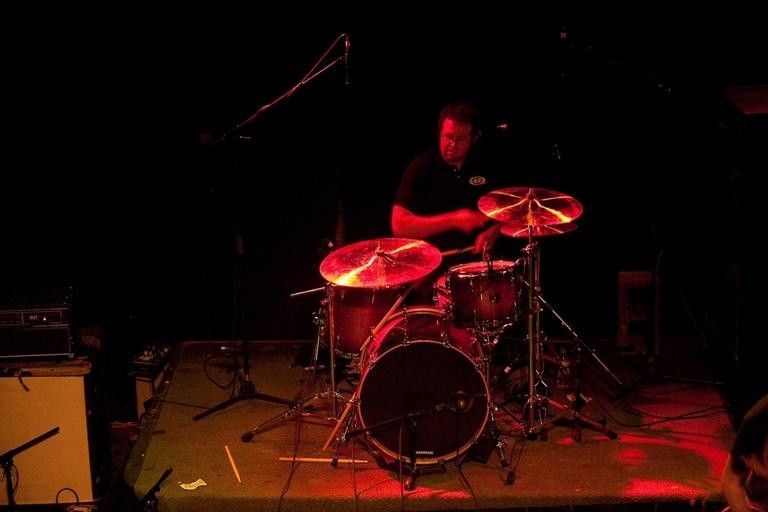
[355,307,491,467]
[446,260,518,330]
[318,283,407,359]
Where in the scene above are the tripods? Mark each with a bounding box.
[193,228,626,488]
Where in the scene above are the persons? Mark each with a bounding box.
[390,105,506,270]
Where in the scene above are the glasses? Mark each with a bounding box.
[441,132,474,143]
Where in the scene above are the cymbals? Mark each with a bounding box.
[320,237,442,288]
[499,222,577,237]
[478,186,584,228]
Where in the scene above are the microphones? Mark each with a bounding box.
[344,41,355,89]
